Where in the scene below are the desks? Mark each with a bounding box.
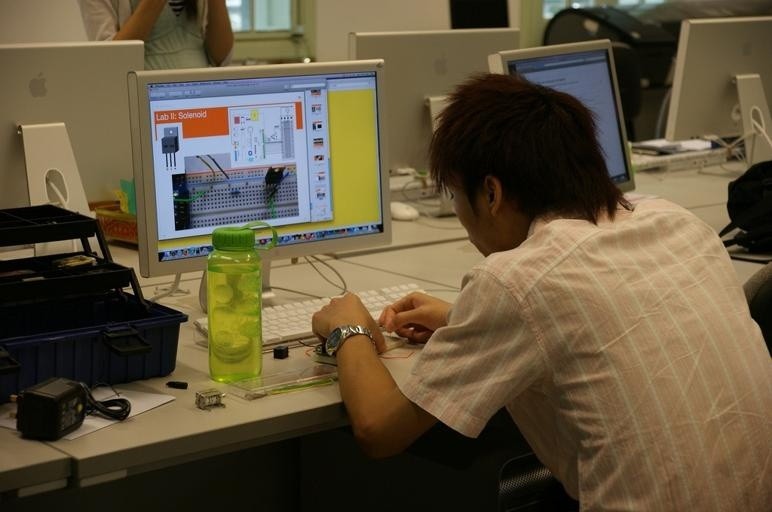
[2,162,769,493]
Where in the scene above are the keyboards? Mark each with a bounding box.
[630,147,728,173]
[389,175,441,201]
[193,283,433,353]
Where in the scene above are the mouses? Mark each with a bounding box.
[390,201,419,221]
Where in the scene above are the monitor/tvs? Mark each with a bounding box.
[348,27,521,217]
[0,40,145,259]
[126,58,392,314]
[487,38,635,193]
[665,15,772,177]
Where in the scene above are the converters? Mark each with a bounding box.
[9,375,86,441]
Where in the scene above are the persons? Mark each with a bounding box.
[75,0,237,72]
[312,70,772,512]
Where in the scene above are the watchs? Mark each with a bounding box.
[325,325,378,359]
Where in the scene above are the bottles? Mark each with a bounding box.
[206,226,261,381]
